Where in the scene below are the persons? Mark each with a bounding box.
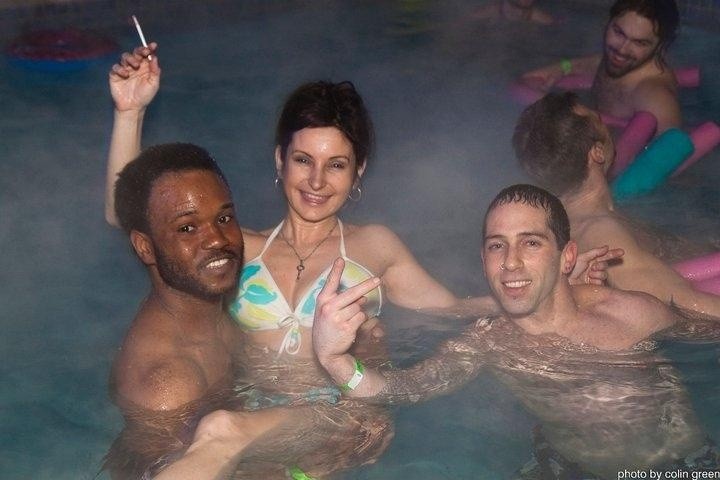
[102,0,720,480]
